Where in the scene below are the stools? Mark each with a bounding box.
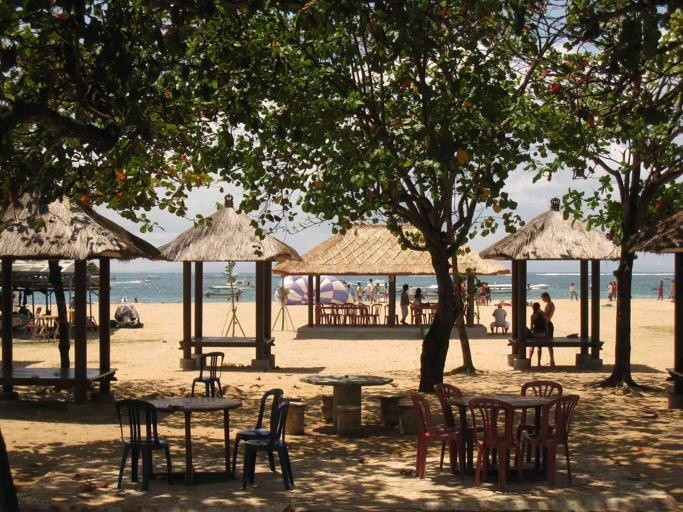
[495,326,504,335]
[285,402,307,436]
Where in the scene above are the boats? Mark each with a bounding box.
[347,279,533,294]
[534,283,553,290]
[207,279,256,289]
[143,275,161,280]
[206,285,243,298]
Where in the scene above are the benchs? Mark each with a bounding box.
[190,336,275,347]
[508,337,602,348]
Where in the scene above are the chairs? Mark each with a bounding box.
[298,374,578,493]
[243,399,295,491]
[191,351,224,399]
[313,298,439,327]
[116,398,172,492]
[230,388,284,475]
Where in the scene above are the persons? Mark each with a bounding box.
[410,287,425,316]
[528,303,547,366]
[489,304,509,334]
[669,279,674,302]
[537,292,555,367]
[399,283,415,324]
[657,280,664,301]
[568,282,578,301]
[350,278,389,303]
[611,280,617,297]
[606,282,612,303]
